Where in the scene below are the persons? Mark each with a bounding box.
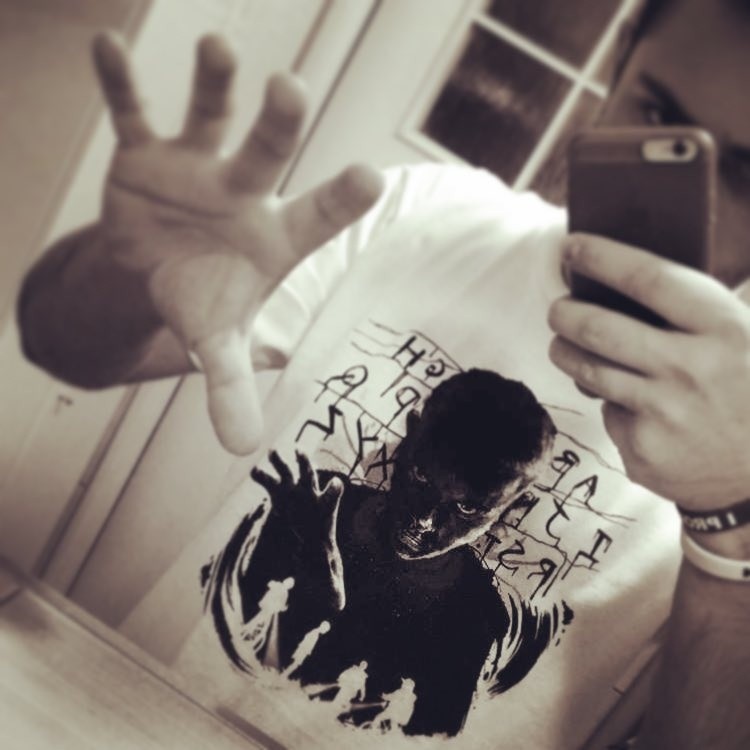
[17,0,750,750]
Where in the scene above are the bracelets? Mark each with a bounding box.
[677,499,750,532]
[681,529,750,581]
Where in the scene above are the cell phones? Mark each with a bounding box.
[564,125,715,399]
